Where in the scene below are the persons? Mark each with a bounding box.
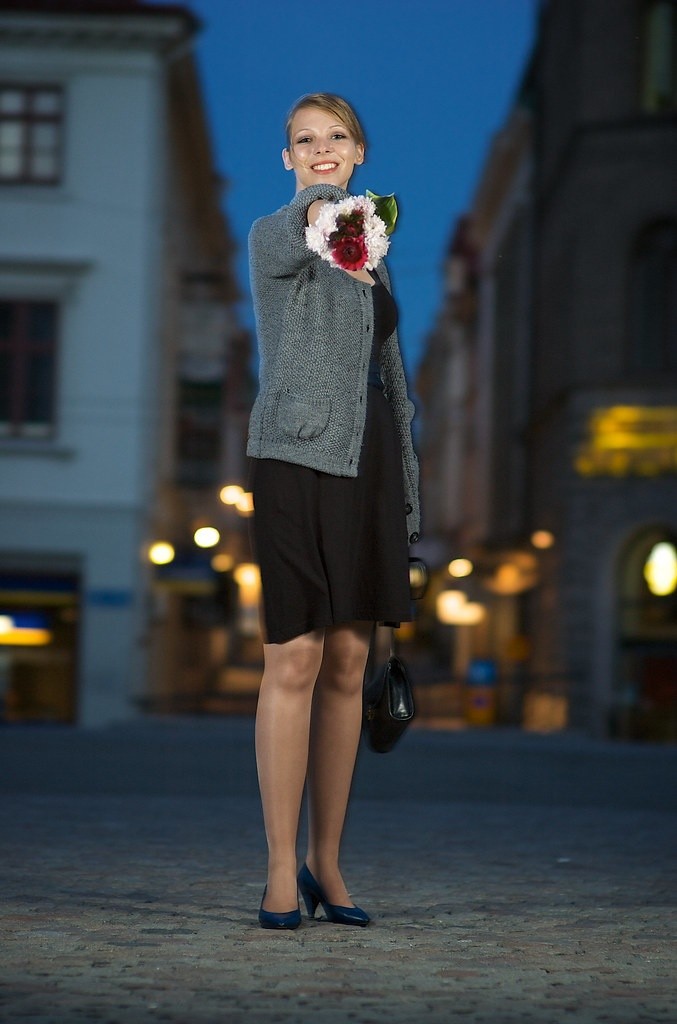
[248,92,420,929]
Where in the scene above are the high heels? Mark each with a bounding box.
[258,884,301,930]
[297,861,371,927]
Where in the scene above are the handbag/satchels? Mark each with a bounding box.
[362,619,414,753]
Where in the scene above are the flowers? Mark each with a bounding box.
[304,188,399,274]
